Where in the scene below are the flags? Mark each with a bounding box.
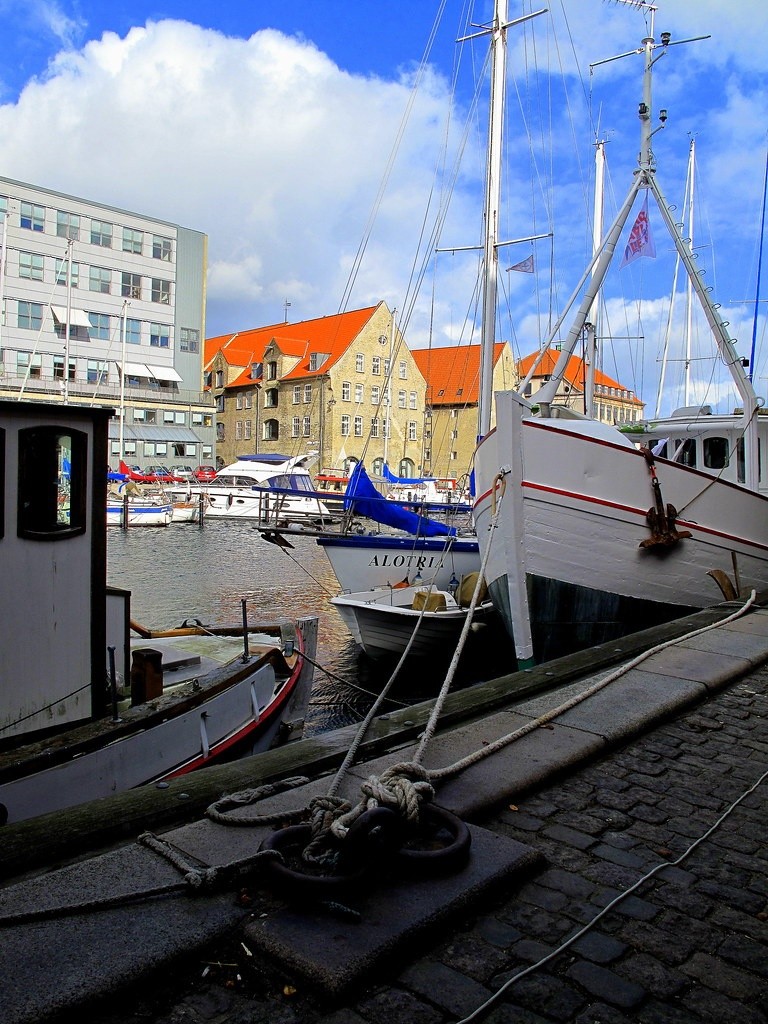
[504,254,534,274]
[617,188,656,270]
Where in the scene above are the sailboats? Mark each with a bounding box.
[60,298,173,526]
[465,0,768,671]
[306,0,621,661]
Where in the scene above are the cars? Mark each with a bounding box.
[167,465,194,483]
[191,465,218,483]
[124,464,144,480]
[142,466,171,483]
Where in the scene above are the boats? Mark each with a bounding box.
[2,232,309,835]
[169,497,212,523]
[159,450,330,525]
